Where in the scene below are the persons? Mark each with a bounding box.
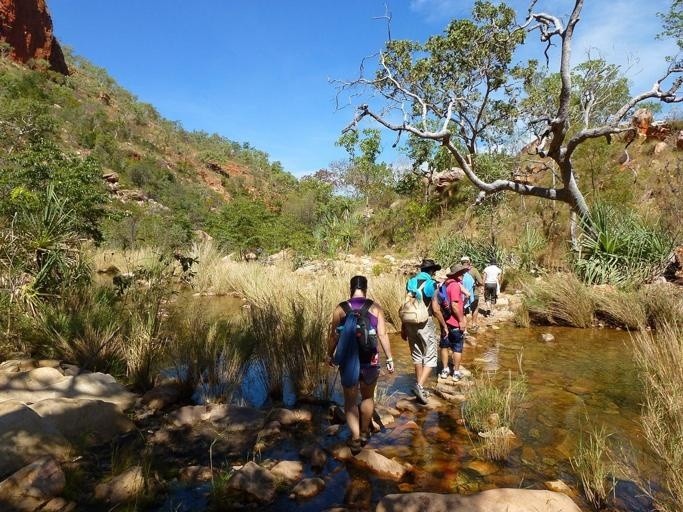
[326,276,394,452]
[401,255,482,404]
[482,256,502,314]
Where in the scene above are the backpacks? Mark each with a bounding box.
[431,279,456,319]
[336,299,379,369]
[398,280,427,324]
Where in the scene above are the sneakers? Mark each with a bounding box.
[452,370,465,382]
[410,385,429,405]
[441,368,450,378]
[346,435,368,455]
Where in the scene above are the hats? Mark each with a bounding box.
[490,257,497,265]
[460,256,472,265]
[421,259,441,271]
[446,262,469,277]
